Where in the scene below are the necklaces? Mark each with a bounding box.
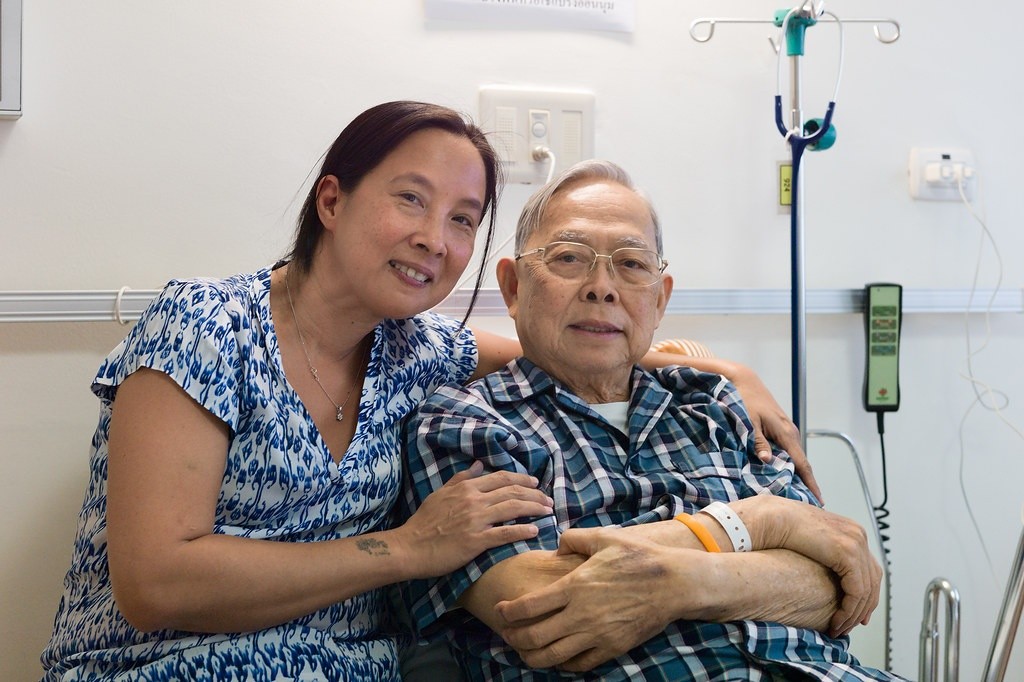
[286,277,367,421]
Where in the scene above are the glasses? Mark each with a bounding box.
[513,241,668,286]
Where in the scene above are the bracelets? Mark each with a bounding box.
[673,512,721,552]
[701,502,752,552]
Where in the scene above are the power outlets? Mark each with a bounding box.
[908,147,980,203]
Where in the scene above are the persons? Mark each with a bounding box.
[41,102,825,682]
[401,162,901,682]
